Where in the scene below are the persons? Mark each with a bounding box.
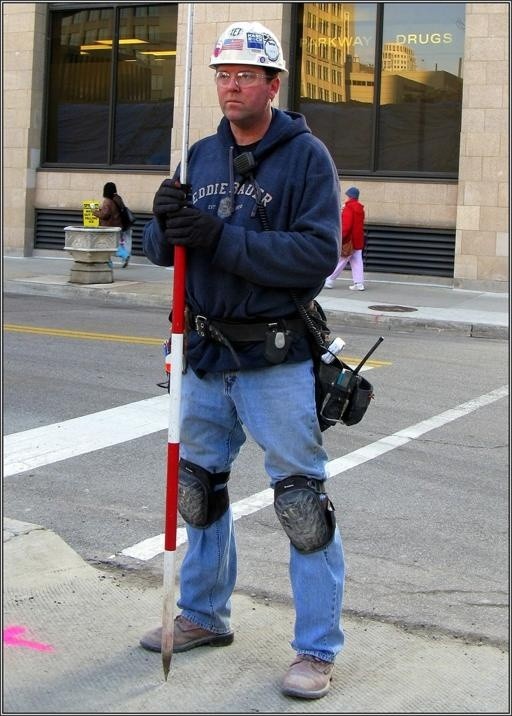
[91,180,132,270]
[322,186,367,291]
[131,18,348,704]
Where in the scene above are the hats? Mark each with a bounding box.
[345,187,359,198]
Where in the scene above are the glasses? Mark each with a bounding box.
[214,70,267,90]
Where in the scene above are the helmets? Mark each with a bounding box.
[208,21,290,80]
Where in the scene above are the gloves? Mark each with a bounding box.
[151,176,193,232]
[166,201,225,261]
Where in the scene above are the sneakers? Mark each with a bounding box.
[349,283,365,290]
[122,255,130,268]
[323,283,332,288]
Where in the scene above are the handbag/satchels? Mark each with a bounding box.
[121,205,135,232]
[341,240,354,257]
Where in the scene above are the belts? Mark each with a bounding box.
[183,312,288,343]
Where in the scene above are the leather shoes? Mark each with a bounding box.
[283,652,334,699]
[140,614,235,653]
